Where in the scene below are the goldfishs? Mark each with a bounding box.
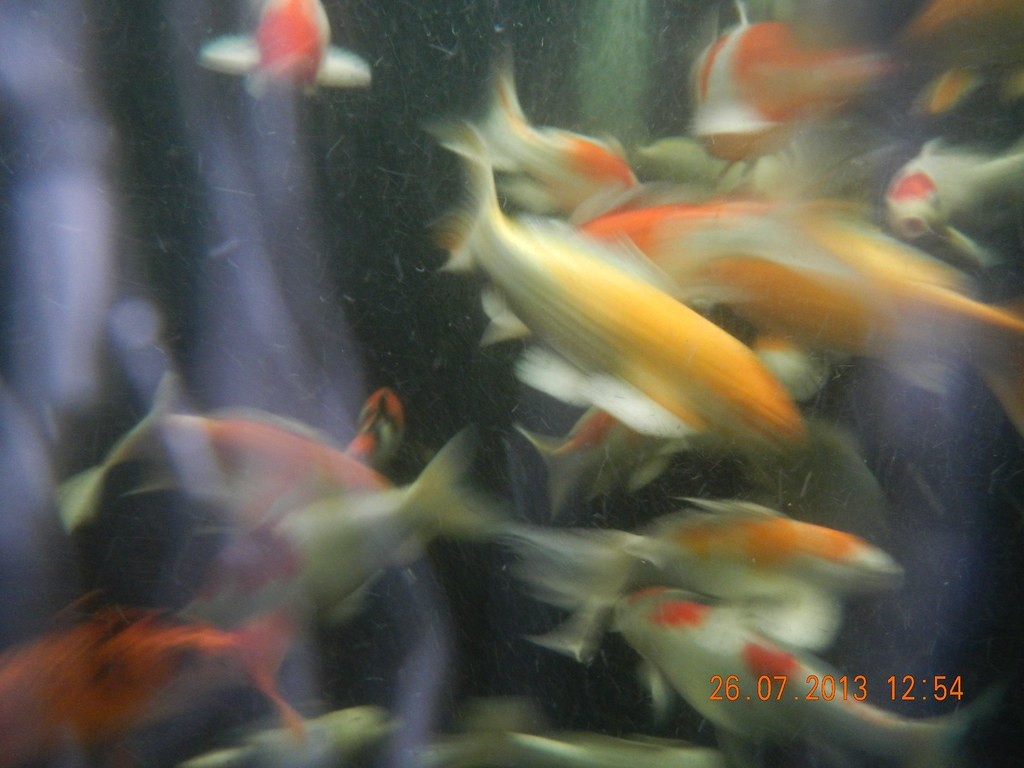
[193,1,372,97]
[418,0,1024,768]
[0,368,527,768]
